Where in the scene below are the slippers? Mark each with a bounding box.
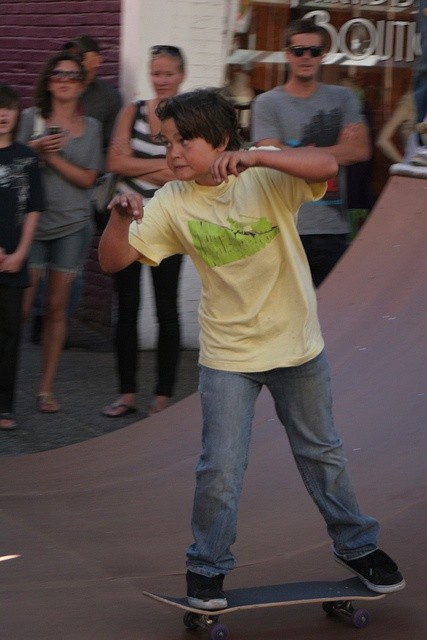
[35,392,61,412]
[145,405,161,417]
[99,400,137,417]
[0,412,17,429]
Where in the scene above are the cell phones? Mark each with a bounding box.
[43,125,68,159]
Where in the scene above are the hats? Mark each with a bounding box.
[62,35,99,51]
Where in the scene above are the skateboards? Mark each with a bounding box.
[141,576,388,639]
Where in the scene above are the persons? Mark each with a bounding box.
[16,54,106,413]
[250,25,369,286]
[103,45,187,418]
[97,89,407,609]
[66,34,124,138]
[0,86,42,431]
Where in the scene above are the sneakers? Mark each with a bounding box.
[185,572,226,609]
[333,549,405,592]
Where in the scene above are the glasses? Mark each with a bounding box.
[288,45,322,57]
[73,51,84,61]
[149,45,179,55]
[49,70,82,79]
